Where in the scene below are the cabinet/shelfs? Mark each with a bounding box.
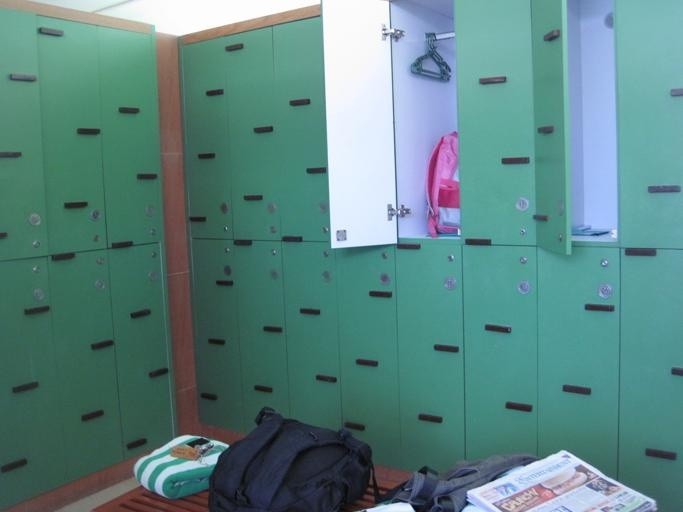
[176,1,682,511]
[1,2,177,512]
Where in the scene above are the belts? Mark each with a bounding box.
[413,465,454,512]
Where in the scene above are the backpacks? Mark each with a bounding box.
[425,131,459,239]
[207,408,372,511]
